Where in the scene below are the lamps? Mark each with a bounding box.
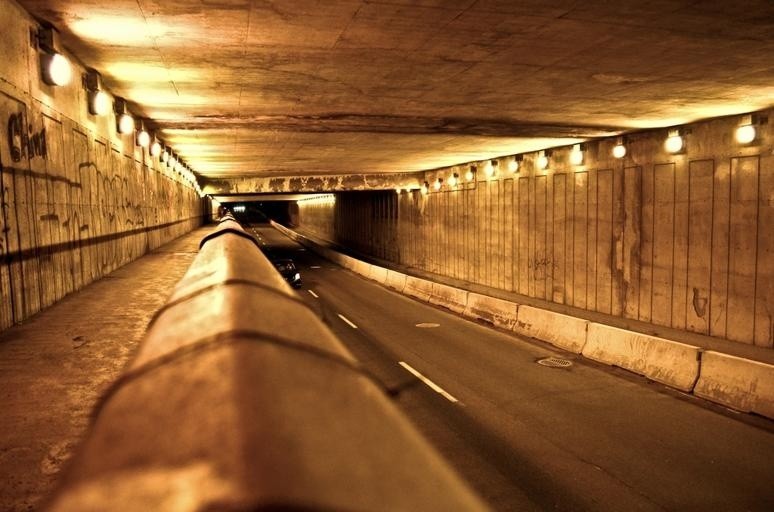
[117,113,134,135]
[149,142,161,156]
[41,53,73,86]
[160,151,169,162]
[135,129,150,147]
[90,91,111,115]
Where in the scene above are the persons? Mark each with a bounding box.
[286,263,293,270]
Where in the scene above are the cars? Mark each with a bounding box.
[271,258,302,290]
